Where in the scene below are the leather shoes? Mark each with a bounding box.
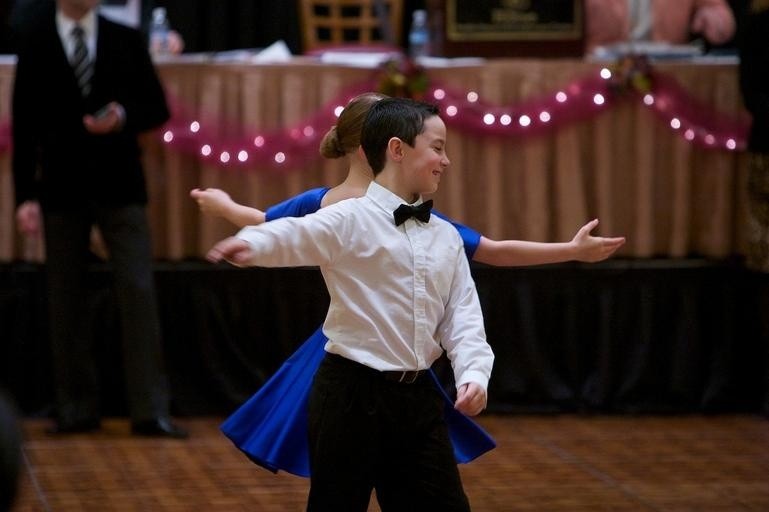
[44,414,103,435]
[130,413,188,438]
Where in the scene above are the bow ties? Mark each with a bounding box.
[393,199,433,227]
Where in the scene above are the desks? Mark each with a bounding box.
[1,52,744,262]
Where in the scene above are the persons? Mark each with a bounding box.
[10,0,191,440]
[192,91,626,478]
[0,199,52,512]
[207,98,495,512]
[734,1,768,277]
[584,0,738,64]
[141,1,304,57]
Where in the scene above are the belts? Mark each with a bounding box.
[396,369,423,386]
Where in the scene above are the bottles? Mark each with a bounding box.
[147,8,171,63]
[406,11,430,69]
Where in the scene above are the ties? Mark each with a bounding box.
[69,25,93,94]
